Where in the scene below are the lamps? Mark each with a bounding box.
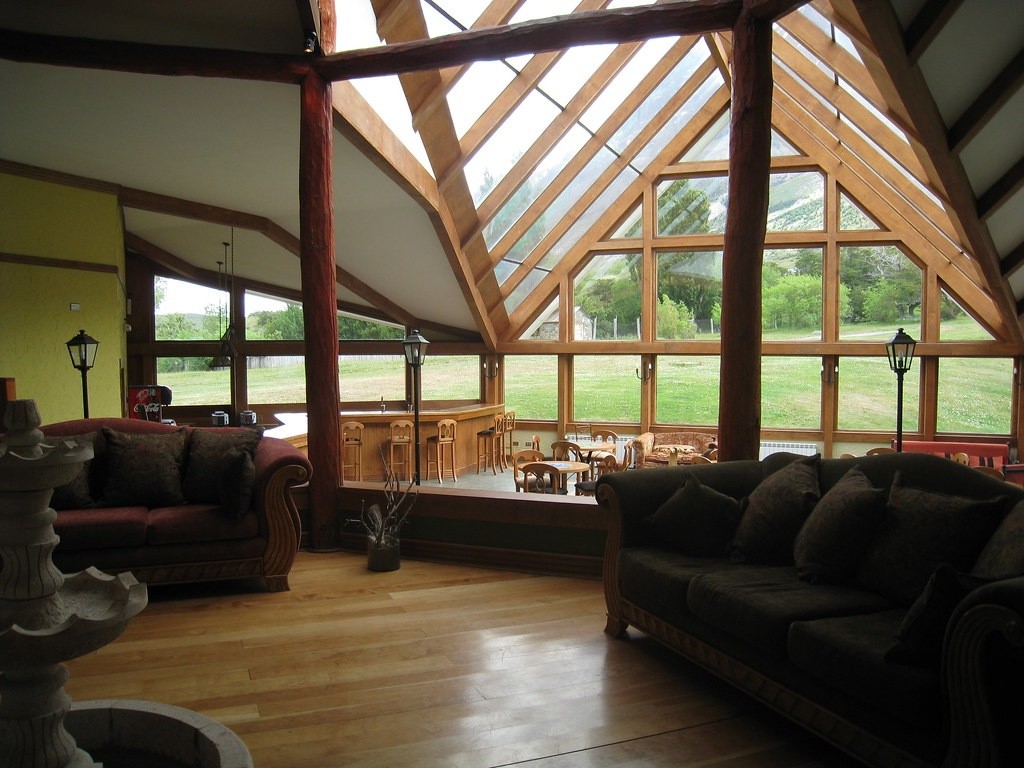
[1013,368,1024,385]
[820,366,839,385]
[636,364,653,382]
[304,31,316,53]
[483,362,499,378]
[208,261,231,368]
[220,240,231,341]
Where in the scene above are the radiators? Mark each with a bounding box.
[565,434,634,469]
[760,443,816,462]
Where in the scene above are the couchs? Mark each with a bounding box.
[632,432,719,468]
[598,450,1024,768]
[38,413,314,594]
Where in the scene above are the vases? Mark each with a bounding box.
[367,544,400,572]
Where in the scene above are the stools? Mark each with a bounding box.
[342,421,364,481]
[474,412,516,474]
[425,418,458,485]
[385,419,415,485]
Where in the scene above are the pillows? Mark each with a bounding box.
[217,447,258,526]
[885,563,994,667]
[736,451,822,562]
[792,464,886,582]
[642,469,745,559]
[101,425,188,508]
[861,472,1010,612]
[43,431,97,510]
[184,425,263,500]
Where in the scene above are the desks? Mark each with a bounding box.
[569,440,616,482]
[518,460,590,488]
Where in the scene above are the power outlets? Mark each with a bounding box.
[513,441,519,446]
[526,442,531,446]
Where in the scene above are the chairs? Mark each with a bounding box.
[512,430,634,497]
